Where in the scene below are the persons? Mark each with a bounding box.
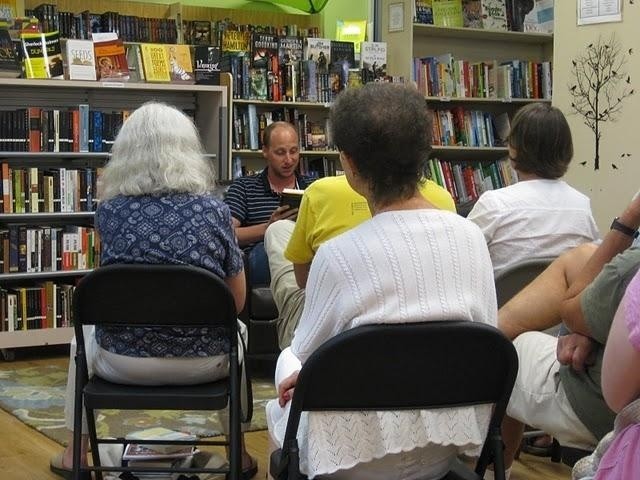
[264,80,500,478]
[498,186,640,478]
[222,119,321,286]
[48,99,259,480]
[568,265,640,478]
[464,101,603,280]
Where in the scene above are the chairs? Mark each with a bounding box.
[270,322,519,480]
[72,264,246,479]
[493,256,571,463]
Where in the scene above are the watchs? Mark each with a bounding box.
[609,216,639,240]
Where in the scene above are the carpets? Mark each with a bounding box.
[0,353,274,451]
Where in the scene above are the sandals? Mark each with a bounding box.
[526,434,554,457]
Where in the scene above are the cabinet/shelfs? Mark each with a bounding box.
[382,1,553,204]
[229,28,382,191]
[1,80,228,360]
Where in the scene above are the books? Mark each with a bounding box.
[0,276,83,332]
[414,0,554,33]
[0,162,104,213]
[413,53,552,99]
[280,187,305,222]
[0,222,104,274]
[232,101,343,151]
[429,106,514,147]
[423,155,522,208]
[182,18,406,104]
[181,107,195,123]
[121,426,199,480]
[230,153,345,189]
[0,3,222,85]
[0,104,129,152]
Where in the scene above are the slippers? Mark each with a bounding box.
[50,449,92,480]
[225,453,258,480]
